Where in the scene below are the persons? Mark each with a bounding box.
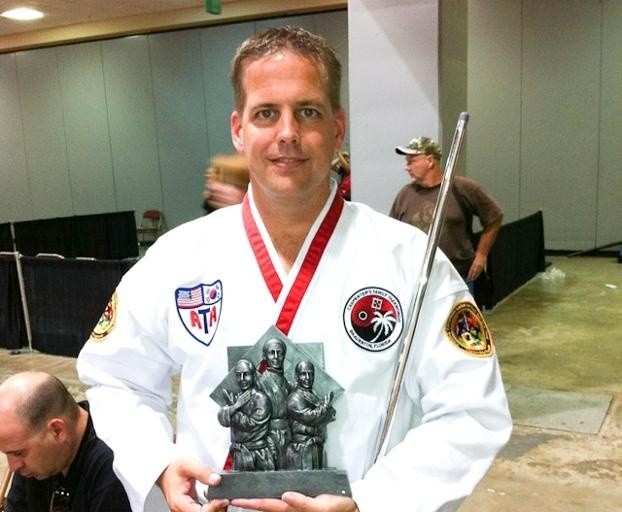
[284,359,338,470]
[76,22,513,511]
[388,136,505,297]
[219,356,274,471]
[205,152,250,210]
[258,335,294,470]
[0,369,133,512]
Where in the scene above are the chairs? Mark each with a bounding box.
[131,210,164,250]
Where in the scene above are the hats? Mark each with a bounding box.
[396,136,443,158]
